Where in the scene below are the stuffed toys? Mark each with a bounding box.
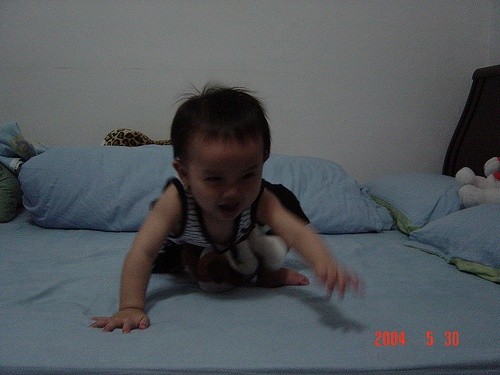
[455,157,500,208]
[100,128,173,148]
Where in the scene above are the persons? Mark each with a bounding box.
[88,80,366,333]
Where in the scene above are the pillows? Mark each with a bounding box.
[0,161,22,223]
[14,144,397,233]
[405,200,500,285]
[362,172,462,236]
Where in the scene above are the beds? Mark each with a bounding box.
[0,219,500,375]
